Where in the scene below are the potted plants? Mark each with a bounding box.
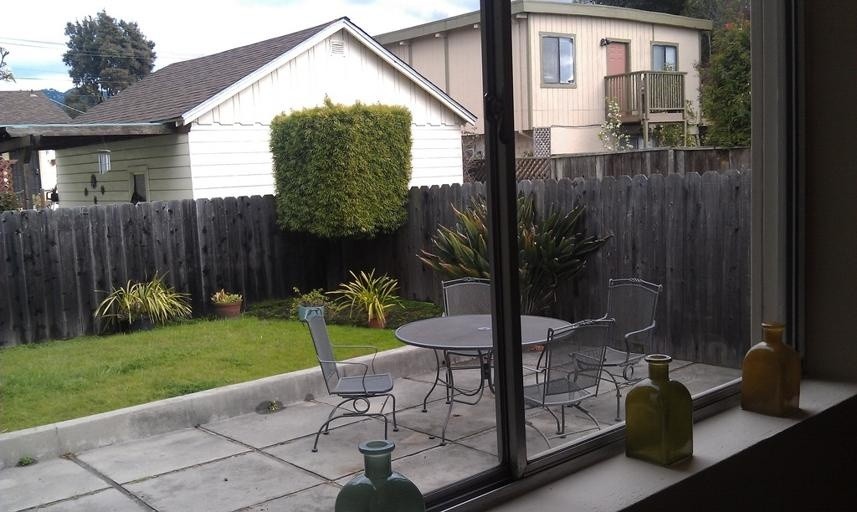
[286,287,338,321]
[211,289,243,319]
[326,269,408,329]
[92,271,193,332]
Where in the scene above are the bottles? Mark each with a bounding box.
[333,439,425,512]
[624,353,694,467]
[740,323,800,418]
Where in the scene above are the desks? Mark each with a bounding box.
[395,315,575,445]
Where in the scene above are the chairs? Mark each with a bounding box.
[523,318,615,449]
[441,277,495,404]
[570,278,663,421]
[304,306,399,451]
